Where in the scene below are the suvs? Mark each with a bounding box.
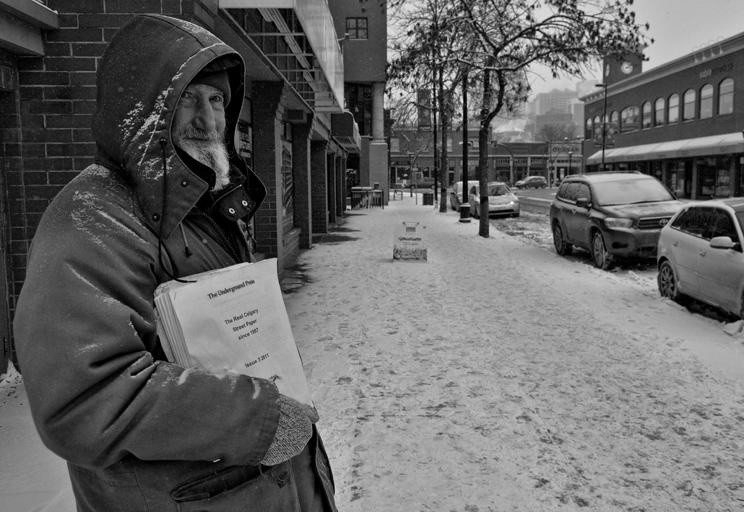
[549,170,685,269]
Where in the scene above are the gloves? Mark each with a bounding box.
[260,394,319,466]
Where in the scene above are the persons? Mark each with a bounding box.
[7,12,341,512]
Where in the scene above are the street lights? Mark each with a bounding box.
[458,55,472,223]
[569,151,573,174]
[596,84,607,165]
[432,36,447,209]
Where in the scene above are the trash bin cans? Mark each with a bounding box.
[423,192,434,205]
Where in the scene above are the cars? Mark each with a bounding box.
[514,175,548,189]
[655,197,744,320]
[450,180,520,218]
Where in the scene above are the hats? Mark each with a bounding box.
[187,57,231,110]
